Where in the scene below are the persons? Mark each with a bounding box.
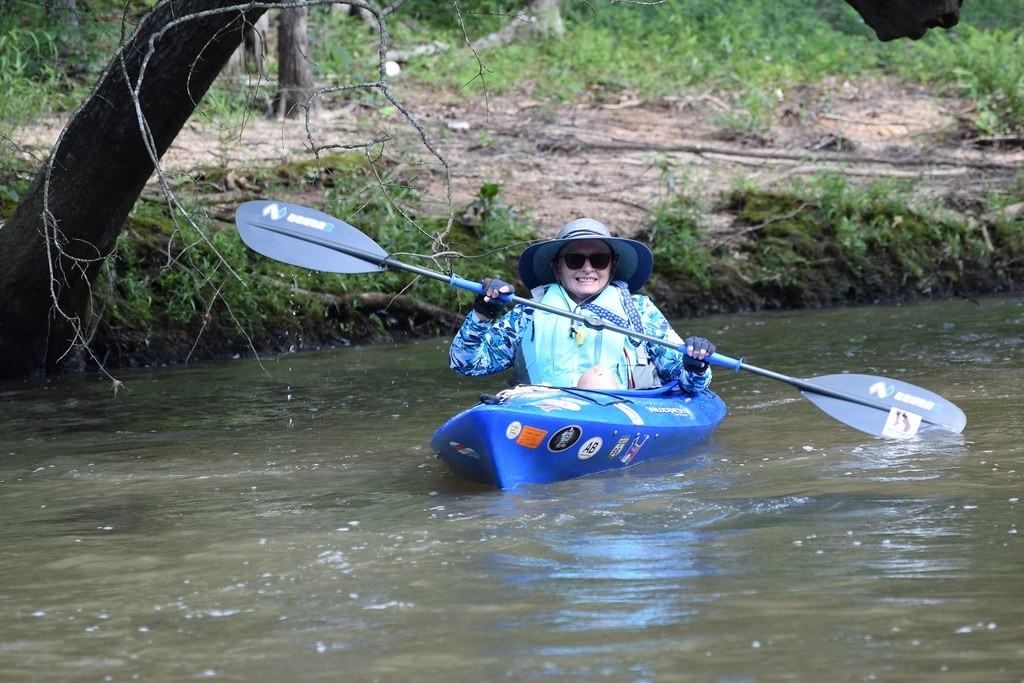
[447,218,716,394]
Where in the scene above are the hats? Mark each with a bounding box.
[518,217,654,294]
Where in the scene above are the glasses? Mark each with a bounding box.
[556,253,619,270]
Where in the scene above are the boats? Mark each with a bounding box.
[430,384,727,489]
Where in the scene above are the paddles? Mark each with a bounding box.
[234,199,968,438]
